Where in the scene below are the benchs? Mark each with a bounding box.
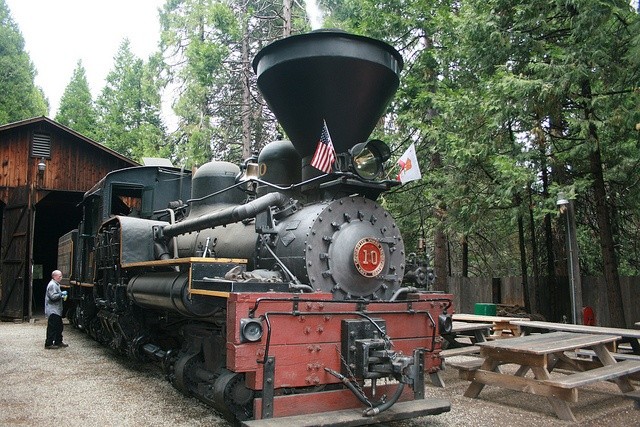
[537,360,637,420]
[429,345,480,388]
[573,348,640,366]
[445,356,503,380]
[437,340,479,359]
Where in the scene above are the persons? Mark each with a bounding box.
[561,314,570,323]
[582,305,595,326]
[45,270,68,350]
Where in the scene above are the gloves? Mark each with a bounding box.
[62,290,67,296]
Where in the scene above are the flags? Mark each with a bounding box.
[384,142,423,186]
[310,119,342,175]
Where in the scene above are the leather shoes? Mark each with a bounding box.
[45,344,59,348]
[54,342,68,346]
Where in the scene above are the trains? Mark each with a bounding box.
[56,30,454,422]
